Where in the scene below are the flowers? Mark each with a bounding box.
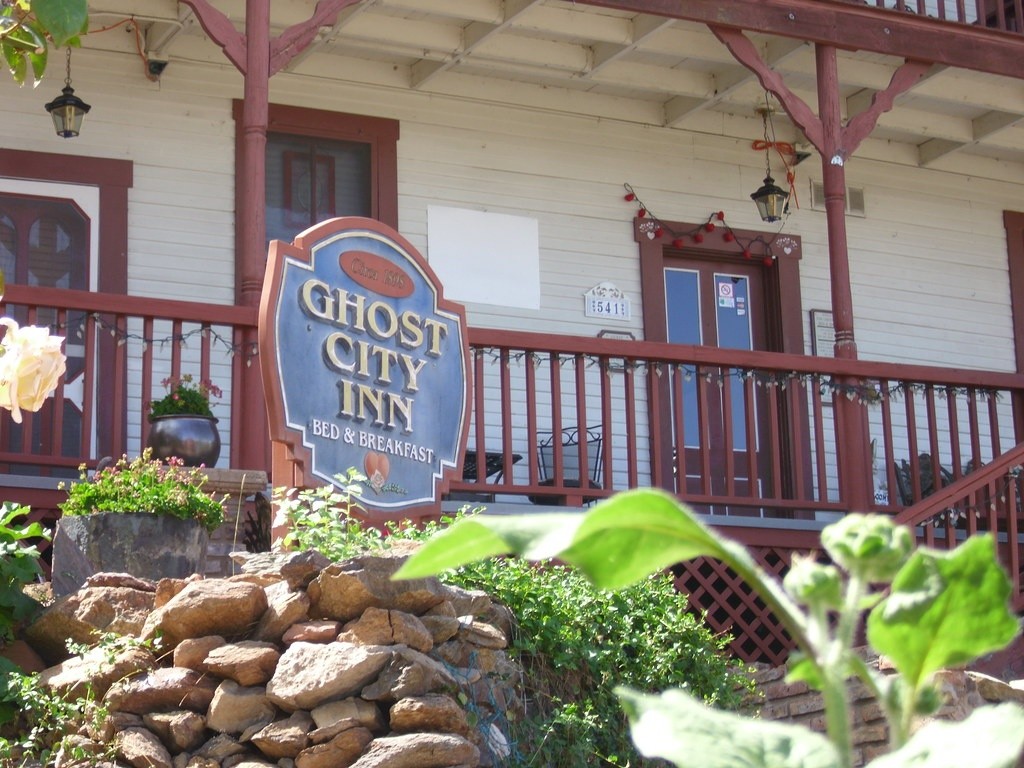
[148,374,226,420]
[56,448,227,532]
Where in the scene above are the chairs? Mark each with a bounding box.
[527,421,610,507]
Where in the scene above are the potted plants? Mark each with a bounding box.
[144,414,223,471]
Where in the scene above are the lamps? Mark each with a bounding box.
[751,103,790,224]
[44,42,91,139]
